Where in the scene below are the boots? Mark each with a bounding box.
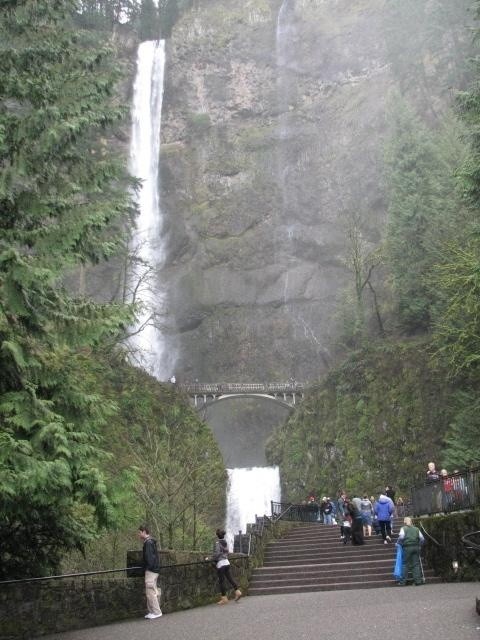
[234,589,242,601]
[217,596,229,605]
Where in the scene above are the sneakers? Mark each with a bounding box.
[147,612,162,619]
[144,613,151,619]
[386,535,391,543]
[384,540,388,545]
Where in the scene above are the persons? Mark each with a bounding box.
[171,375,176,384]
[393,517,425,586]
[306,485,404,545]
[204,529,242,605]
[167,377,171,383]
[424,461,470,517]
[137,524,163,619]
